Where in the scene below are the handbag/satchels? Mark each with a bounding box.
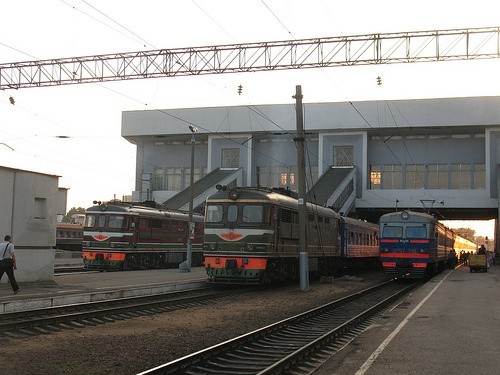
[1,259,13,271]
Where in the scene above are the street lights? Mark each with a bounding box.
[186,124,199,271]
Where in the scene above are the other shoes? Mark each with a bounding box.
[14,288,22,294]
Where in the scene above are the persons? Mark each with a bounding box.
[0,235,23,295]
[448,244,495,270]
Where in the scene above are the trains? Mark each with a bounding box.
[201,183,380,287]
[56,223,83,251]
[80,200,205,272]
[378,210,481,278]
